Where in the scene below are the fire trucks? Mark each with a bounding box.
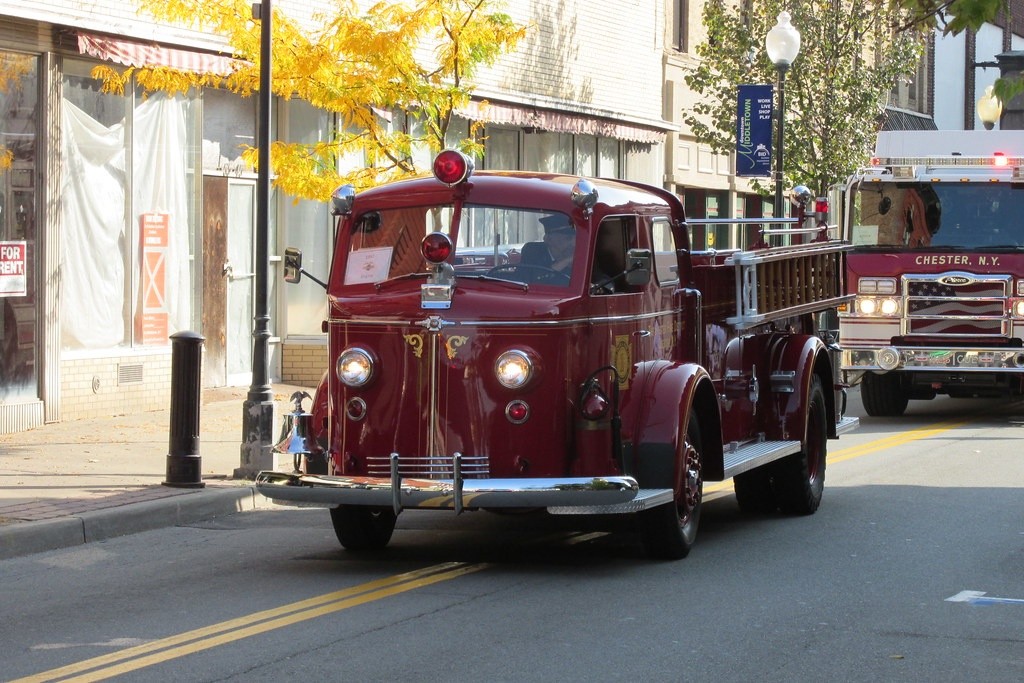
[791,126,1024,417]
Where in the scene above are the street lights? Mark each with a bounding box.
[763,10,801,247]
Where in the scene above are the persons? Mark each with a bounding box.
[510,214,615,295]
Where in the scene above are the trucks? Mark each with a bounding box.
[253,145,855,562]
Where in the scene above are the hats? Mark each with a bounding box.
[537,214,576,237]
